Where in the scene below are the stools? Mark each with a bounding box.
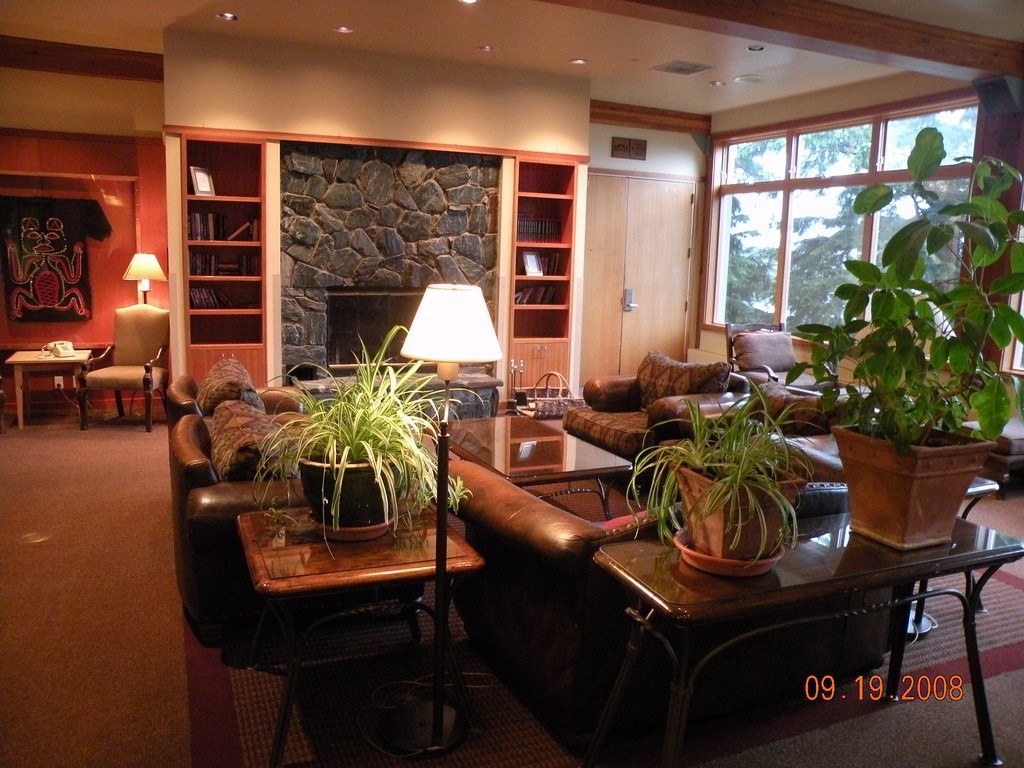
[958,417,1024,501]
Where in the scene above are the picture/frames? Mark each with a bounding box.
[189,166,216,196]
[520,250,544,276]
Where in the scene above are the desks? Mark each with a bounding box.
[906,476,1000,640]
[592,513,1024,768]
[832,386,913,417]
[5,350,93,430]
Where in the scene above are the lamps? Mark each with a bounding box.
[122,252,168,305]
[399,281,502,768]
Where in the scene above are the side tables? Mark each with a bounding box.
[233,508,490,768]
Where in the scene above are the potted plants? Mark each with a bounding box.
[786,127,1024,552]
[250,323,487,562]
[625,378,827,577]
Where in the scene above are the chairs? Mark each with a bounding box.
[725,323,825,391]
[78,304,170,433]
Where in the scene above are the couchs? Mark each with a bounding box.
[167,375,304,436]
[562,350,751,498]
[444,430,893,768]
[169,400,331,649]
[704,380,842,458]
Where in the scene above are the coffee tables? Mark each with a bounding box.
[424,416,633,521]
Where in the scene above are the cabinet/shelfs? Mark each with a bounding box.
[180,135,267,391]
[507,157,579,402]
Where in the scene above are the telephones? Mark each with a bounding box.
[46,340,75,357]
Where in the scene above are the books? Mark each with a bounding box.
[186,213,262,309]
[515,214,562,304]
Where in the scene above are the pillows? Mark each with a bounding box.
[748,382,823,432]
[210,400,285,482]
[195,358,267,415]
[734,330,796,372]
[636,350,731,414]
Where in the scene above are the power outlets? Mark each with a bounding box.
[54,376,64,389]
